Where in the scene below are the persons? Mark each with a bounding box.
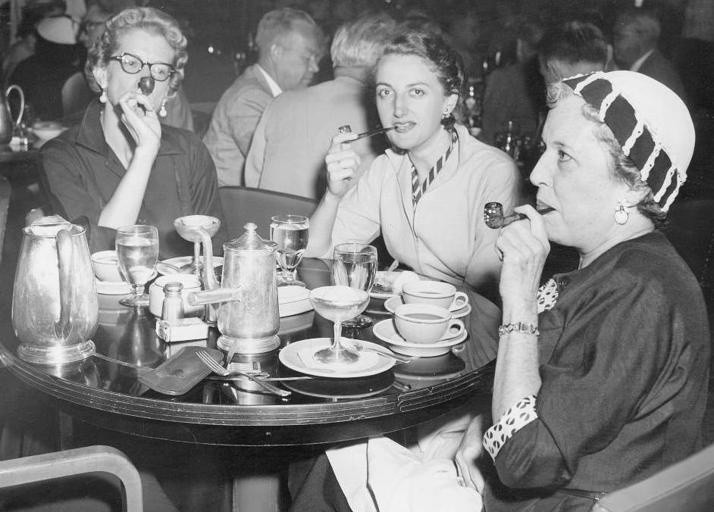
[38,7,229,261]
[203,8,321,189]
[610,11,688,111]
[481,24,559,164]
[537,22,612,110]
[244,13,402,201]
[62,2,194,135]
[1,0,82,123]
[284,70,713,511]
[306,29,520,295]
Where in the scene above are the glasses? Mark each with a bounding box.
[107,52,180,83]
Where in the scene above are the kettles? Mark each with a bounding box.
[188,223,282,342]
[11,207,99,368]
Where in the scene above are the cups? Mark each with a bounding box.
[89,250,128,280]
[269,213,309,286]
[116,225,160,309]
[174,215,220,275]
[493,119,519,160]
[332,243,379,331]
[393,302,464,344]
[401,281,469,312]
[306,285,372,368]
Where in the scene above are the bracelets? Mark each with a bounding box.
[496,321,540,336]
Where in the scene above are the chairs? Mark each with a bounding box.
[0,445,144,512]
[219,186,318,241]
[593,443,714,512]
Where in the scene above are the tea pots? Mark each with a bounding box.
[0,84,26,145]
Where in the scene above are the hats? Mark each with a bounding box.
[562,71,696,211]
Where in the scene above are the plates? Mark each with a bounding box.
[383,294,473,321]
[367,270,421,299]
[277,335,399,380]
[94,276,136,297]
[277,284,318,319]
[372,318,468,359]
[155,255,224,278]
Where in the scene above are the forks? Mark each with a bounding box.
[330,337,410,365]
[194,348,291,399]
